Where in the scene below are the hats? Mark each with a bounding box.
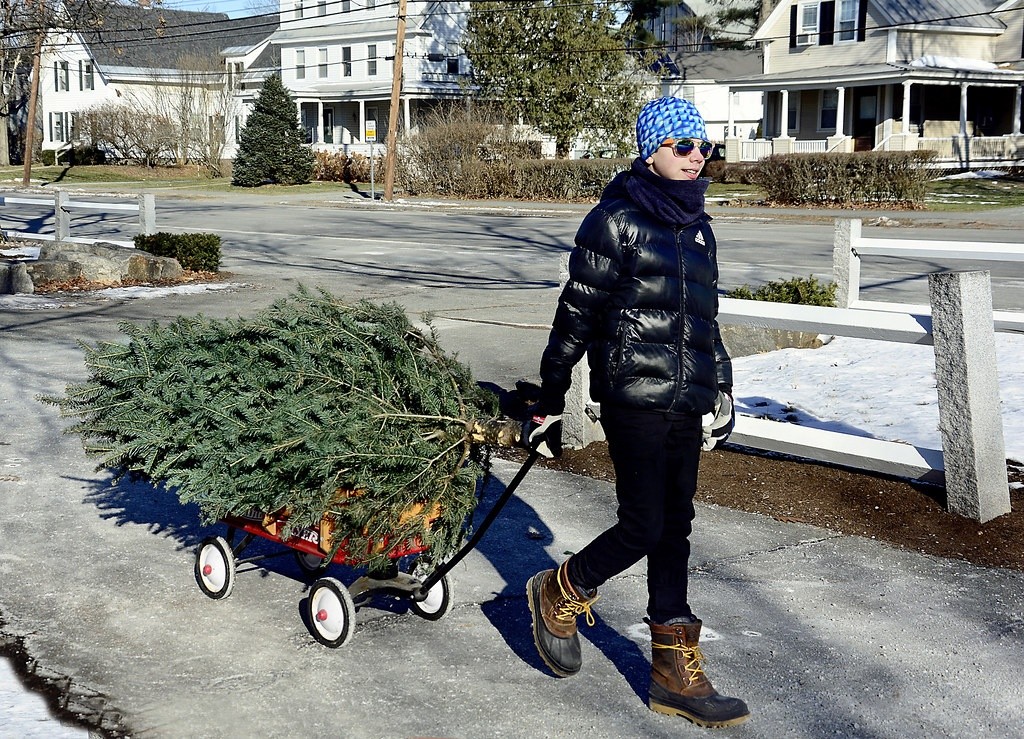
[636,95,708,161]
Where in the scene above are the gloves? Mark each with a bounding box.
[521,412,564,459]
[702,388,740,450]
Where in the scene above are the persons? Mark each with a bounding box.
[526,96,750,727]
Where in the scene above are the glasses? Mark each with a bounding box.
[660,139,712,158]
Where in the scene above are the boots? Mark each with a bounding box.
[642,617,752,728]
[526,554,600,678]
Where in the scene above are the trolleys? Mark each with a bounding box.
[193,409,539,654]
